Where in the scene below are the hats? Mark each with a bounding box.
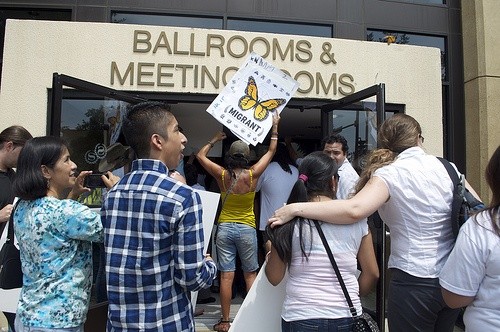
[227,140,250,158]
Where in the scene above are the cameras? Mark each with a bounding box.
[84,174,108,188]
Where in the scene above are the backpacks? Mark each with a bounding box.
[436,156,488,244]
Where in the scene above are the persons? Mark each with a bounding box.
[268,114,481,332]
[320,135,362,203]
[195,108,280,332]
[9,136,121,332]
[263,150,379,331]
[98,103,217,332]
[439,144,500,332]
[0,126,36,332]
[245,135,300,266]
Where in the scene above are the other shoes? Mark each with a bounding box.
[195,296,216,303]
[194,307,204,317]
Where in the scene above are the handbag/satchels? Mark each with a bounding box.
[0,197,23,289]
[351,306,381,332]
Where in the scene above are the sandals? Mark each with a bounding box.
[213,318,230,332]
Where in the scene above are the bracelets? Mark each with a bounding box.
[208,141,213,148]
[271,131,279,135]
[270,138,279,140]
